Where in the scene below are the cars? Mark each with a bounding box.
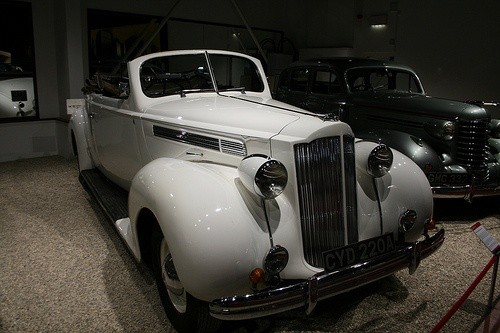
[0,52,40,120]
[271,56,500,208]
[66,49,447,333]
